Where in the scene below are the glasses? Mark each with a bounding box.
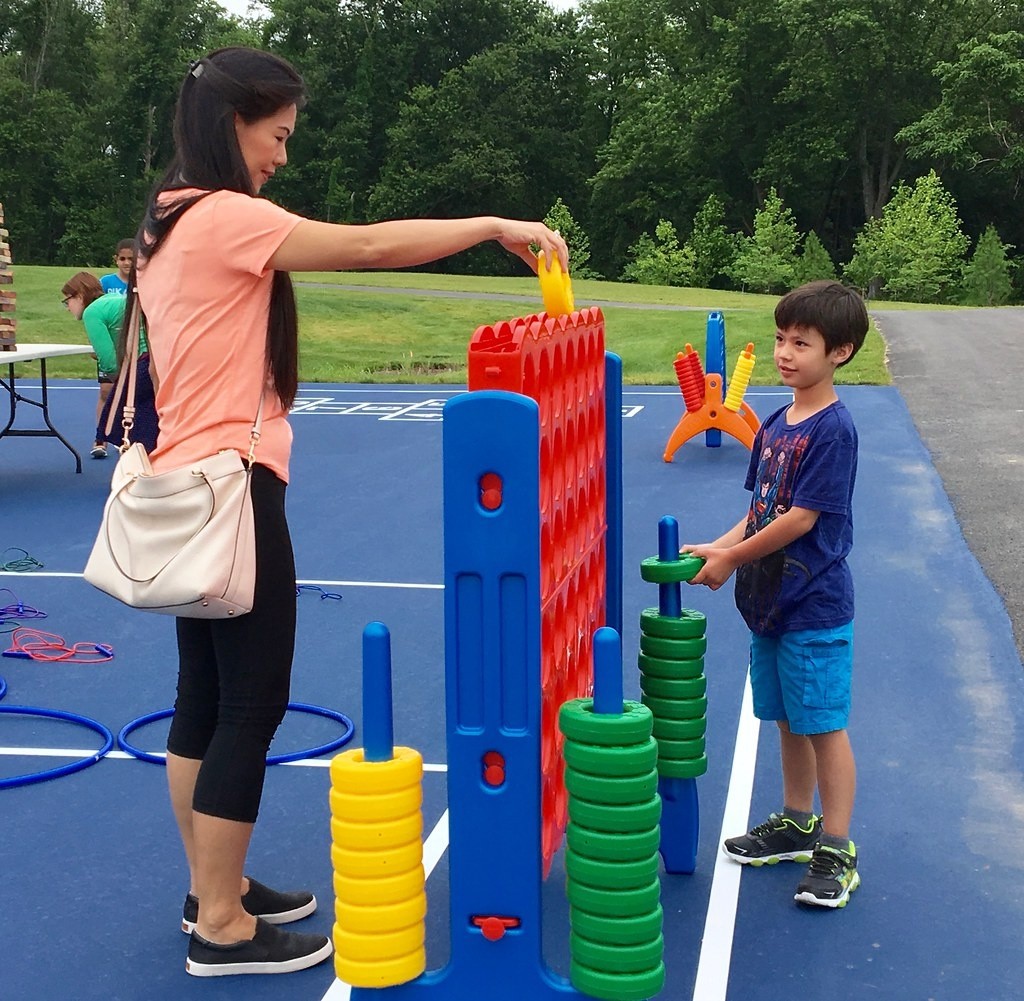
[61,295,74,307]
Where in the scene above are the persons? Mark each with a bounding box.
[134,44,569,974]
[678,280,869,904]
[89,239,134,457]
[60,272,161,456]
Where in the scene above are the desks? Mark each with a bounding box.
[0,343,97,473]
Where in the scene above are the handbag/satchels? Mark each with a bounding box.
[94,353,158,455]
[81,442,256,620]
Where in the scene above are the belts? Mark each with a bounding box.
[139,352,149,359]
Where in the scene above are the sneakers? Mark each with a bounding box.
[185,914,333,977]
[90,442,109,457]
[722,812,823,867]
[794,839,861,908]
[181,874,318,935]
